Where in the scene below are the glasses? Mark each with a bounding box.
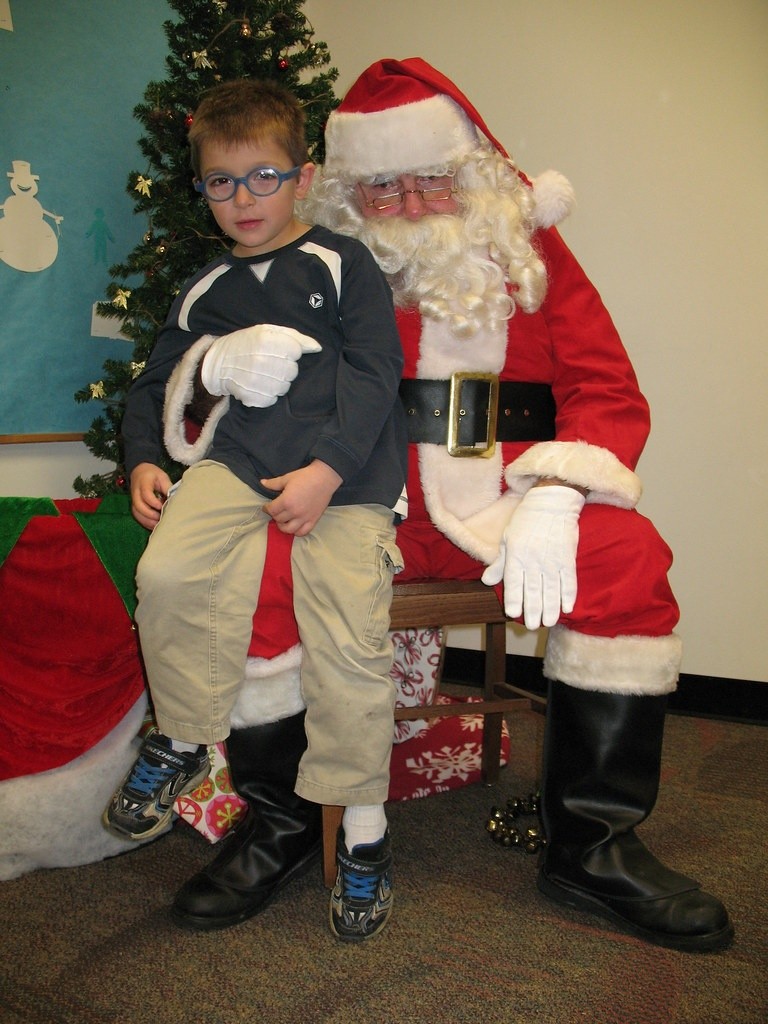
[194,165,299,201]
[357,174,459,211]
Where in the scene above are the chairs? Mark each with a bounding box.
[324,580,550,889]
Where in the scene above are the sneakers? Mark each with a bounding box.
[328,825,393,941]
[101,732,212,839]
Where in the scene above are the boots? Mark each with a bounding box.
[535,623,735,951]
[172,644,322,932]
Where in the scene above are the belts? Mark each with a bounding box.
[398,373,557,458]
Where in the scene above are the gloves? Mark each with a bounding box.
[482,485,587,630]
[202,324,322,406]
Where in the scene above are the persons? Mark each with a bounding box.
[96,82,407,940]
[164,57,734,951]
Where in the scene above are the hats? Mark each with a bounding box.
[320,57,573,230]
